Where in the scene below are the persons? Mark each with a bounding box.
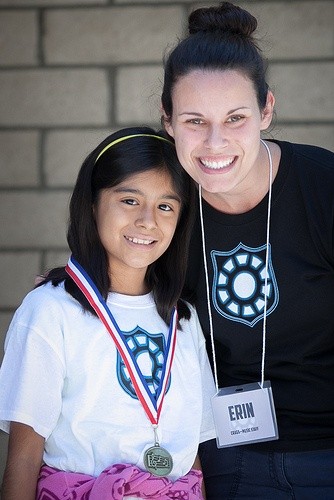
[32,3,334,500]
[0,128,219,500]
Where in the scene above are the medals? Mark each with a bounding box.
[144,445,173,477]
